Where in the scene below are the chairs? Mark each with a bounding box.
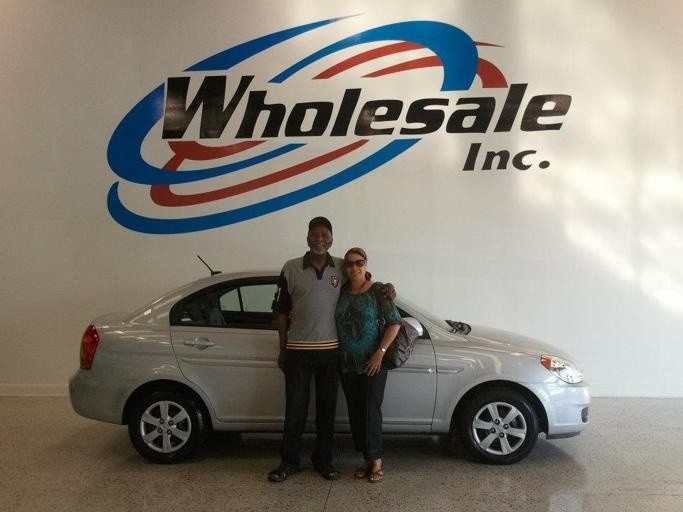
[172,294,228,326]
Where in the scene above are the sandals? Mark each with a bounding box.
[356,463,366,478]
[369,464,386,483]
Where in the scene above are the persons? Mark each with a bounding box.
[287,248,401,482]
[266,217,396,482]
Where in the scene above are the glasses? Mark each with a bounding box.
[345,259,366,267]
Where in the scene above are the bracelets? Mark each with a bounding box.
[377,347,386,353]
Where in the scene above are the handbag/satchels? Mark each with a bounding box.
[373,282,417,369]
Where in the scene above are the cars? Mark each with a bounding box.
[65,253,593,466]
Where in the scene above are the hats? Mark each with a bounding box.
[309,216,332,232]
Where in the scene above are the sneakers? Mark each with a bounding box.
[313,463,341,480]
[267,464,298,482]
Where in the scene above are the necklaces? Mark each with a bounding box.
[347,280,365,314]
